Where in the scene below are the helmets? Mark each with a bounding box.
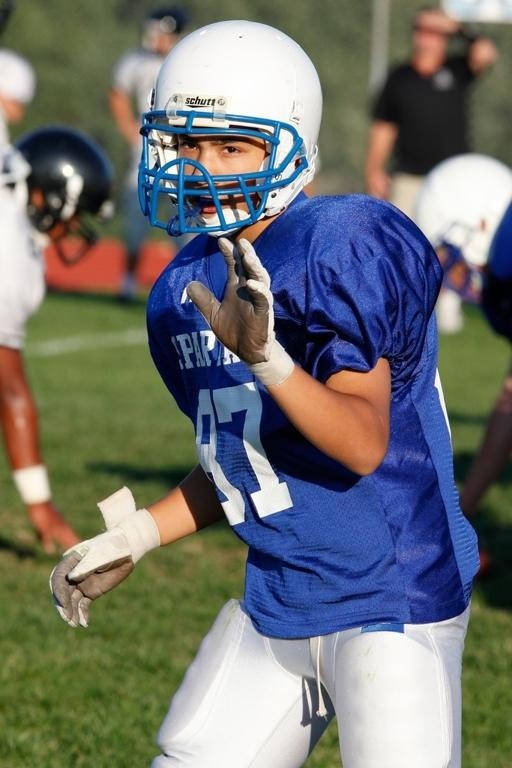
[138,19,322,234]
[2,127,113,266]
[414,152,511,300]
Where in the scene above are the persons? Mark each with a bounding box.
[362,5,498,335]
[414,151,511,509]
[108,2,189,308]
[0,0,38,128]
[46,15,482,768]
[2,118,114,561]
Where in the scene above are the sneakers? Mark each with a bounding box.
[435,290,464,333]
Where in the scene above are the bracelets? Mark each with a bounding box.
[10,461,53,507]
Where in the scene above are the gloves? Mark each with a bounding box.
[185,237,291,385]
[50,483,162,628]
